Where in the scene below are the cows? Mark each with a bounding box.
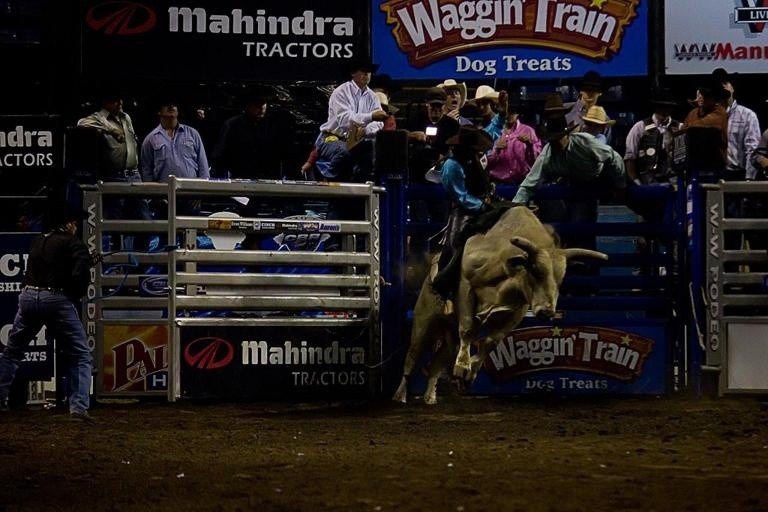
[393,206,608,404]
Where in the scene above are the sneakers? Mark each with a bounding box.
[71,410,95,421]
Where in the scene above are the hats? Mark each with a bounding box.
[538,71,616,141]
[426,79,500,110]
[40,199,90,229]
[649,68,737,106]
[342,59,380,72]
[445,124,494,150]
[232,85,275,108]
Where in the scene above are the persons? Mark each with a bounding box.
[210,89,297,222]
[76,81,161,271]
[142,92,210,276]
[0,202,100,423]
[301,53,767,293]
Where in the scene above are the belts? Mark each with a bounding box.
[24,284,62,290]
[103,169,138,178]
